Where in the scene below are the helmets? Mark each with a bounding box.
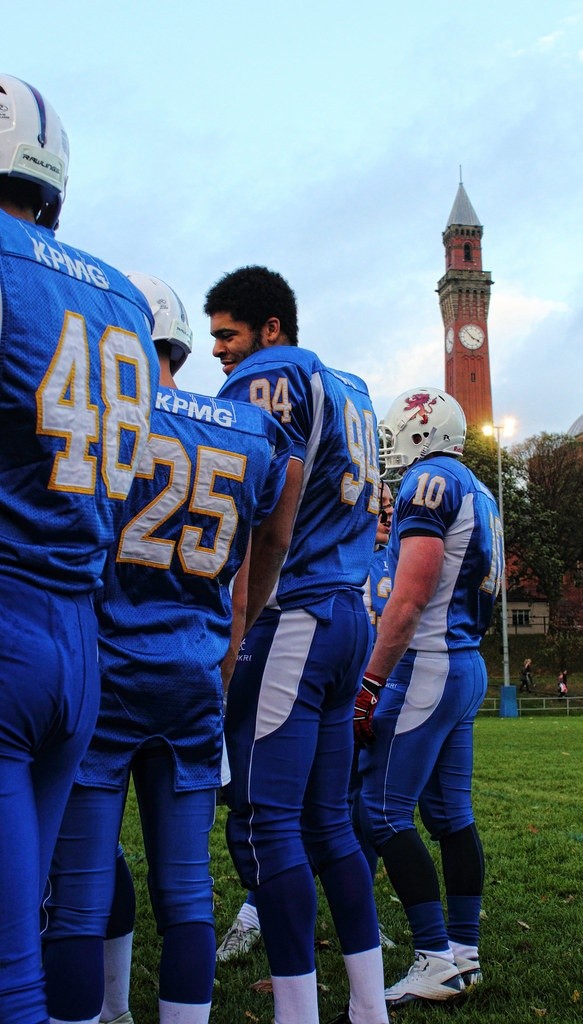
[0,73,70,231]
[122,271,194,378]
[377,385,468,470]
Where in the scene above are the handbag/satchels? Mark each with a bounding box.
[560,683,568,693]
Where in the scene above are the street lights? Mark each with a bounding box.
[481,415,519,718]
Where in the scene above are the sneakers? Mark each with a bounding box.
[383,952,483,1012]
[214,919,262,962]
[98,1010,135,1024]
[378,931,397,952]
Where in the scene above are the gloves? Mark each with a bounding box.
[353,671,387,750]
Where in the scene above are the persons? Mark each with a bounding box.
[354,384,505,1003]
[38,270,290,1024]
[557,668,567,701]
[0,67,166,1024]
[215,608,283,964]
[95,843,136,1023]
[199,263,393,1024]
[519,658,535,693]
[358,475,398,950]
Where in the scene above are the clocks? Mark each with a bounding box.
[458,323,485,350]
[445,327,455,354]
